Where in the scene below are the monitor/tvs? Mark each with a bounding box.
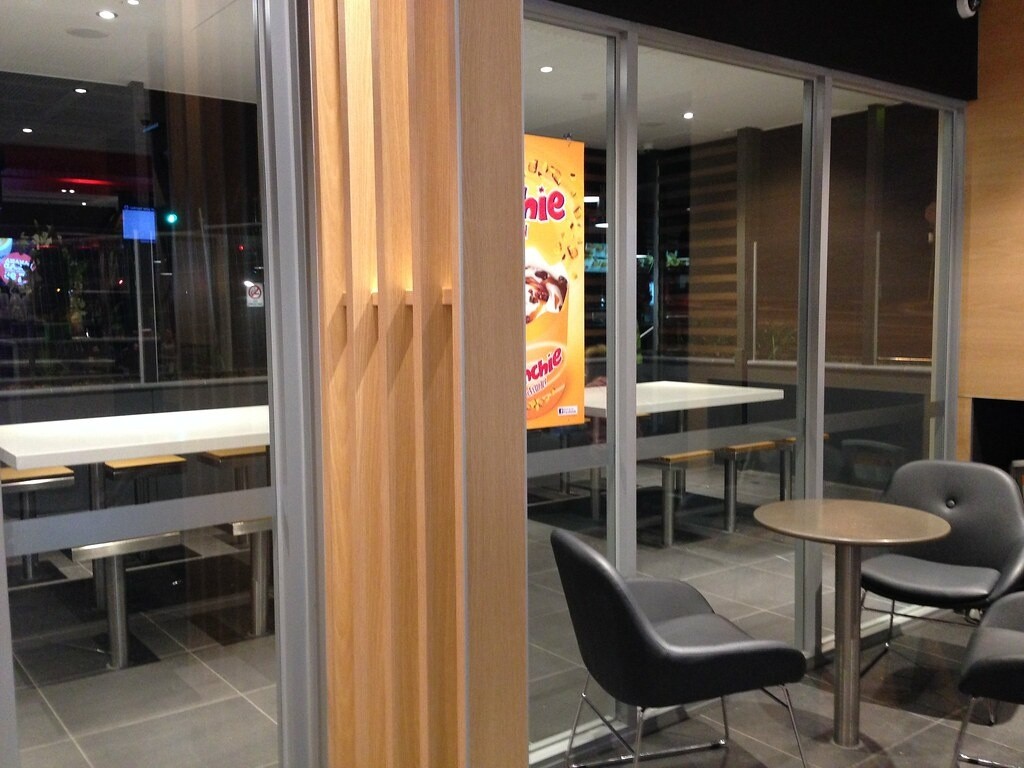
[122,206,156,240]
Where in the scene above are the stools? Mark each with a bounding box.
[716,441,785,533]
[0,464,78,582]
[65,528,186,668]
[101,455,189,564]
[219,518,271,637]
[196,446,265,493]
[637,447,711,548]
[776,434,829,447]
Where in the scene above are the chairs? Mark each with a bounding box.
[861,461,1024,724]
[549,527,809,768]
[949,591,1024,768]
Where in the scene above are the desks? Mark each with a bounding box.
[584,378,784,520]
[753,497,951,750]
[0,405,269,609]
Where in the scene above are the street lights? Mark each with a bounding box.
[165,212,180,381]
